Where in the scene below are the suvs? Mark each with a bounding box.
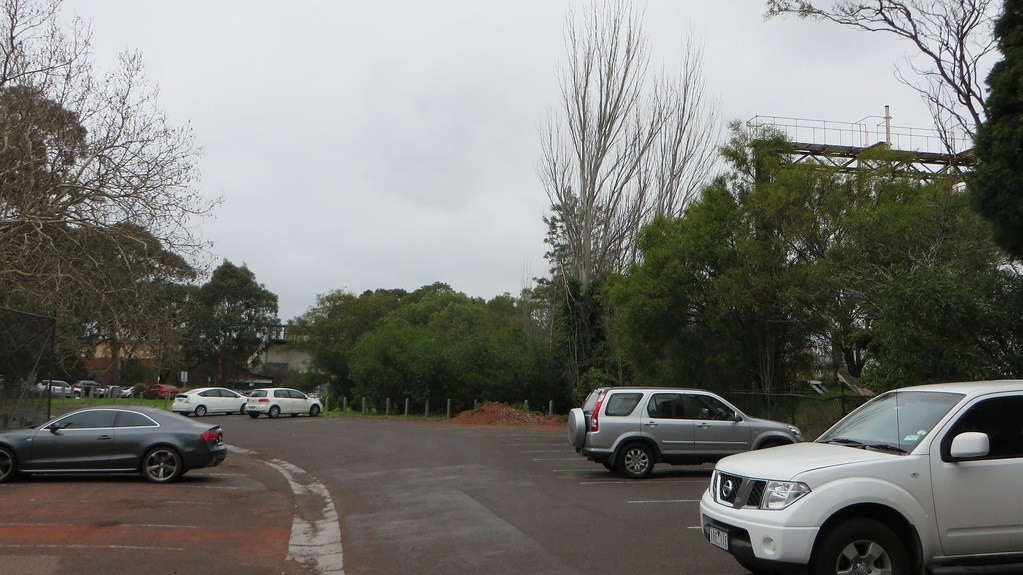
[95,385,122,398]
[246,388,324,418]
[37,380,82,399]
[700,380,1023,575]
[567,386,806,479]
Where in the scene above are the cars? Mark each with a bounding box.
[73,380,102,396]
[146,384,178,399]
[0,405,227,484]
[172,387,247,417]
[120,386,137,398]
